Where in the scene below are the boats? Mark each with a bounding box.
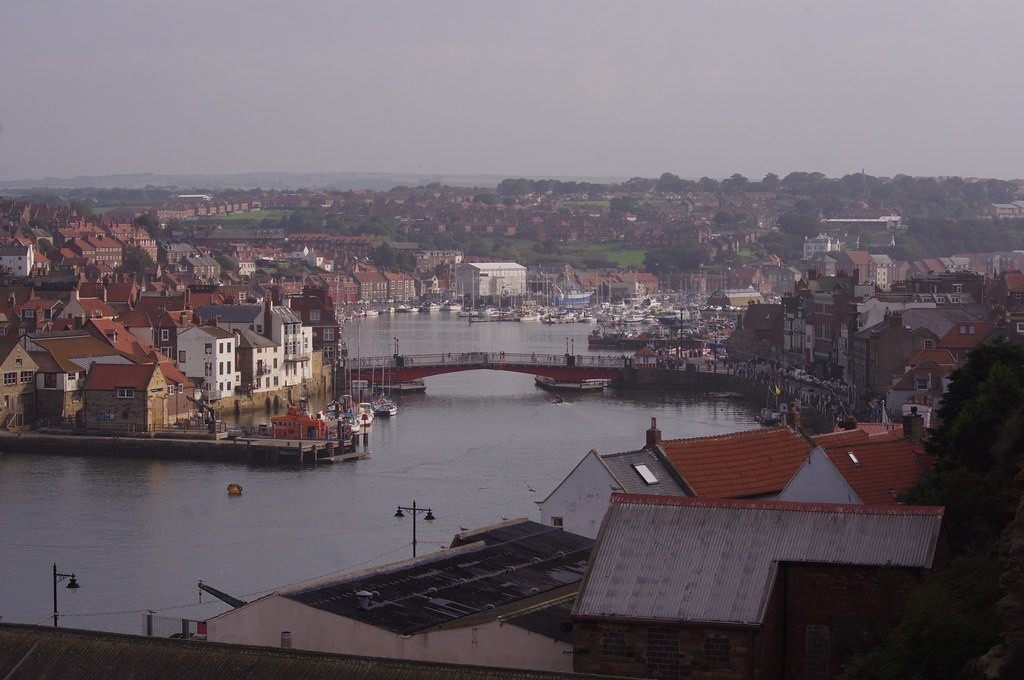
[533,375,612,392]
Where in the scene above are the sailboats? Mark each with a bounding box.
[339,269,690,325]
[314,324,398,435]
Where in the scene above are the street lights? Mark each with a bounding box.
[393,496,434,558]
[53,562,82,627]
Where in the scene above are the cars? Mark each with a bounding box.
[778,367,848,396]
